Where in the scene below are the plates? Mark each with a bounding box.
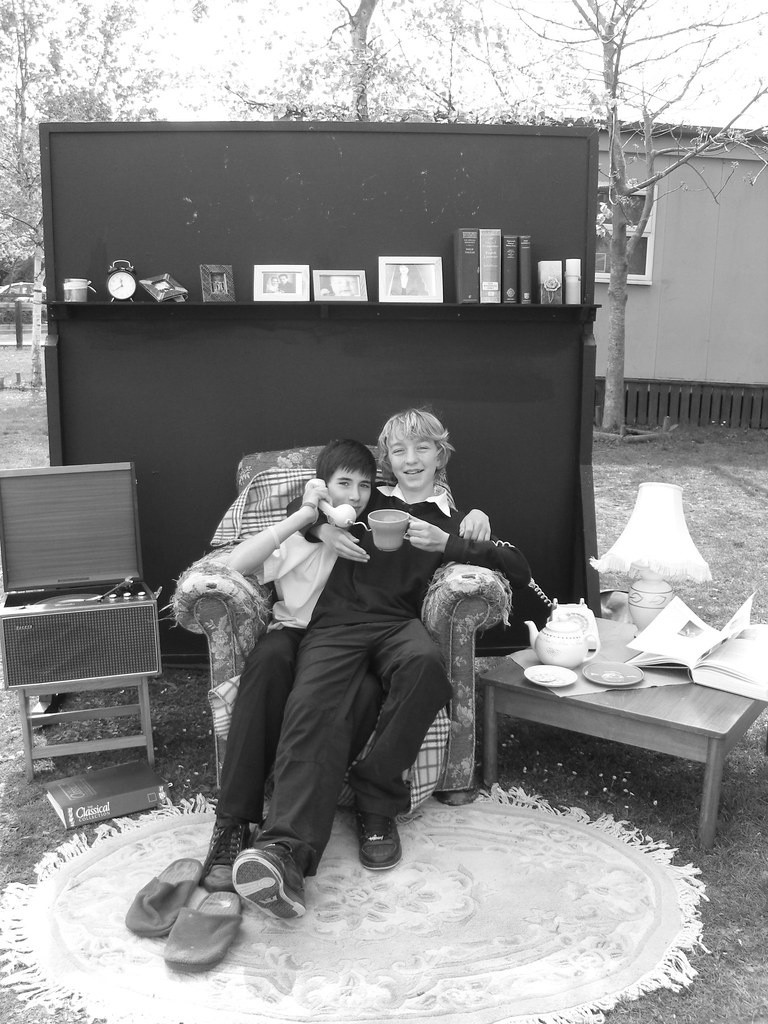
[582,661,644,686]
[524,665,578,687]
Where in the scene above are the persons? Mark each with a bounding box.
[233,403,530,919]
[319,275,357,297]
[200,440,491,895]
[391,265,419,295]
[266,274,296,293]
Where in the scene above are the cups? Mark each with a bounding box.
[367,508,417,552]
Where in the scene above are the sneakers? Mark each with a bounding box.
[197,820,251,892]
[357,809,403,870]
[232,841,307,920]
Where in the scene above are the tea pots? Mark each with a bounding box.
[523,616,601,670]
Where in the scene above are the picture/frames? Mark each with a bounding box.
[138,273,189,303]
[199,264,236,302]
[253,265,311,302]
[313,270,368,302]
[378,256,444,303]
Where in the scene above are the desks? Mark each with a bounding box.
[478,618,768,851]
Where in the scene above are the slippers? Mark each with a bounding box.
[163,890,243,973]
[125,858,203,938]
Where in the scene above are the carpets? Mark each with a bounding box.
[0,781,710,1024]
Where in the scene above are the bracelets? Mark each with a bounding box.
[300,502,319,525]
[269,526,280,549]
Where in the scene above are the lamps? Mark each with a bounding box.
[589,482,712,638]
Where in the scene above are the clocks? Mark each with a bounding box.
[105,258,138,302]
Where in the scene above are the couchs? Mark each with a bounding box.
[157,444,511,807]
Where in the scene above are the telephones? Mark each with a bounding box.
[306,478,357,527]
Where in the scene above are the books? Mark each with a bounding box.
[453,228,532,304]
[623,591,768,701]
[44,759,174,829]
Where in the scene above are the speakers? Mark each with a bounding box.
[0,462,163,691]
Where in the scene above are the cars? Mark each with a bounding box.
[0,282,47,302]
